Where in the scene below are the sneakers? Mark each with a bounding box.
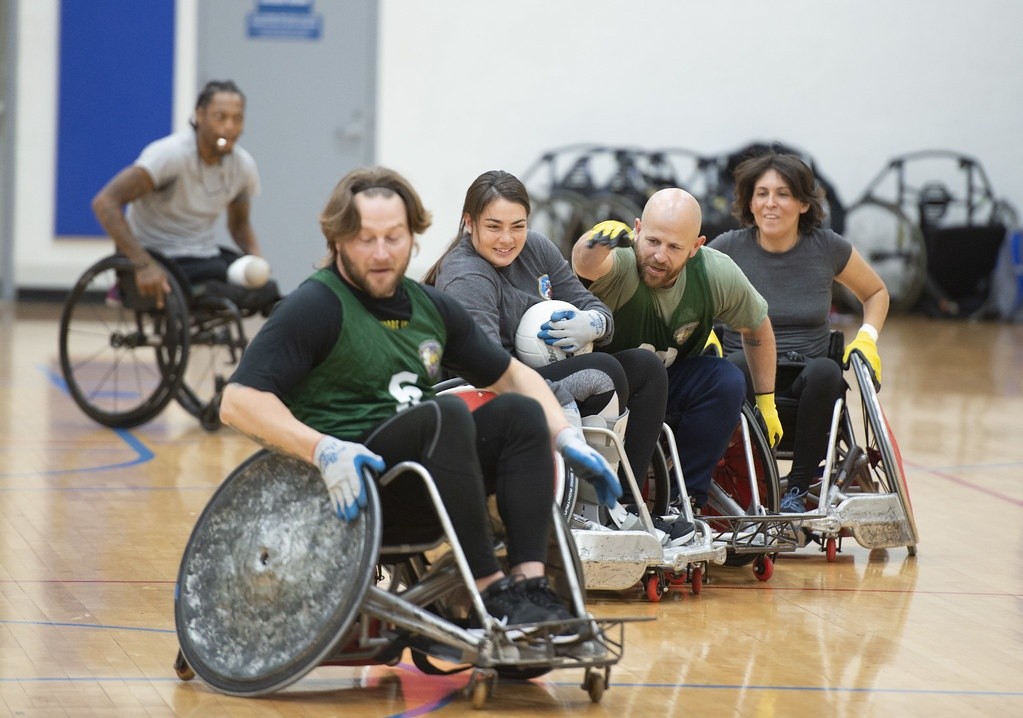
[649,492,708,524]
[445,574,558,639]
[624,504,696,547]
[780,487,808,513]
[513,576,584,643]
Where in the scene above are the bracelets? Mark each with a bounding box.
[859,324,877,343]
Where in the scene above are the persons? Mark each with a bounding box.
[426,171,696,548]
[219,166,619,662]
[707,152,889,513]
[94,79,281,319]
[572,187,784,516]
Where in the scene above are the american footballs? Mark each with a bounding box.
[225,253,272,290]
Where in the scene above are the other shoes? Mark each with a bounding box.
[604,510,673,548]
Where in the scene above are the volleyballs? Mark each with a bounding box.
[514,299,594,369]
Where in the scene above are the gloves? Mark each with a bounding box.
[537,309,607,353]
[753,390,784,456]
[585,219,635,251]
[699,325,724,358]
[560,401,592,443]
[552,427,624,510]
[842,323,883,393]
[312,434,386,523]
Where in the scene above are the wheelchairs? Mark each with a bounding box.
[171,138,1022,711]
[58,245,290,432]
